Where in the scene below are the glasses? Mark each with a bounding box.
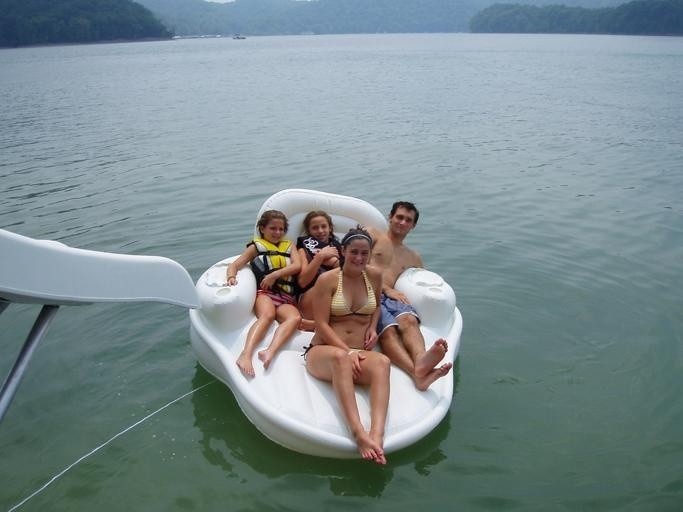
[395,214,413,223]
